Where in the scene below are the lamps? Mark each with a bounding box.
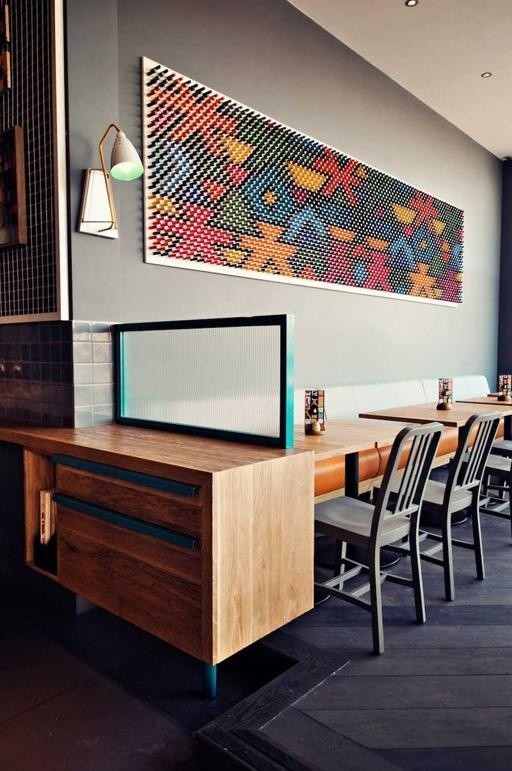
[77,121,144,240]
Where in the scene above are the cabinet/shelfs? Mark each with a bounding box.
[0,421,317,698]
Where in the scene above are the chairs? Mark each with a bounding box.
[450,452,511,547]
[375,414,500,601]
[483,438,512,500]
[313,421,443,652]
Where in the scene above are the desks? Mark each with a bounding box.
[333,417,459,478]
[359,402,511,443]
[456,394,511,407]
[294,417,378,497]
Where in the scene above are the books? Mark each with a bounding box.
[38,487,58,545]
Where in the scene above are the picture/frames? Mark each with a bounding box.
[0,125,28,251]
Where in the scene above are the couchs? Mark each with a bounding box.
[293,374,491,421]
